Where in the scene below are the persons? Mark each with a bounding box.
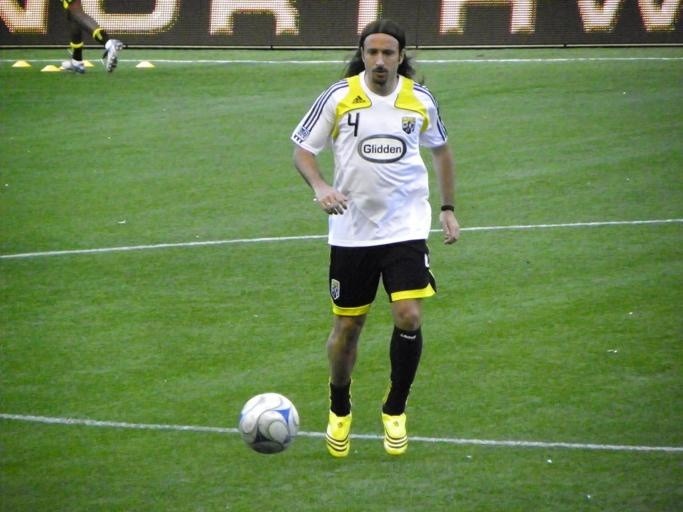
[289,21,461,457]
[59,0,121,74]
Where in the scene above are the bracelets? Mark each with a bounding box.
[441,205,455,211]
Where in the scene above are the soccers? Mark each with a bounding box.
[239,392,300,455]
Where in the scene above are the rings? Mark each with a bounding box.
[326,202,331,206]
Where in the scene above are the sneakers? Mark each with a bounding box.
[381,411,407,454]
[102,39,123,73]
[62,60,85,74]
[325,409,353,458]
